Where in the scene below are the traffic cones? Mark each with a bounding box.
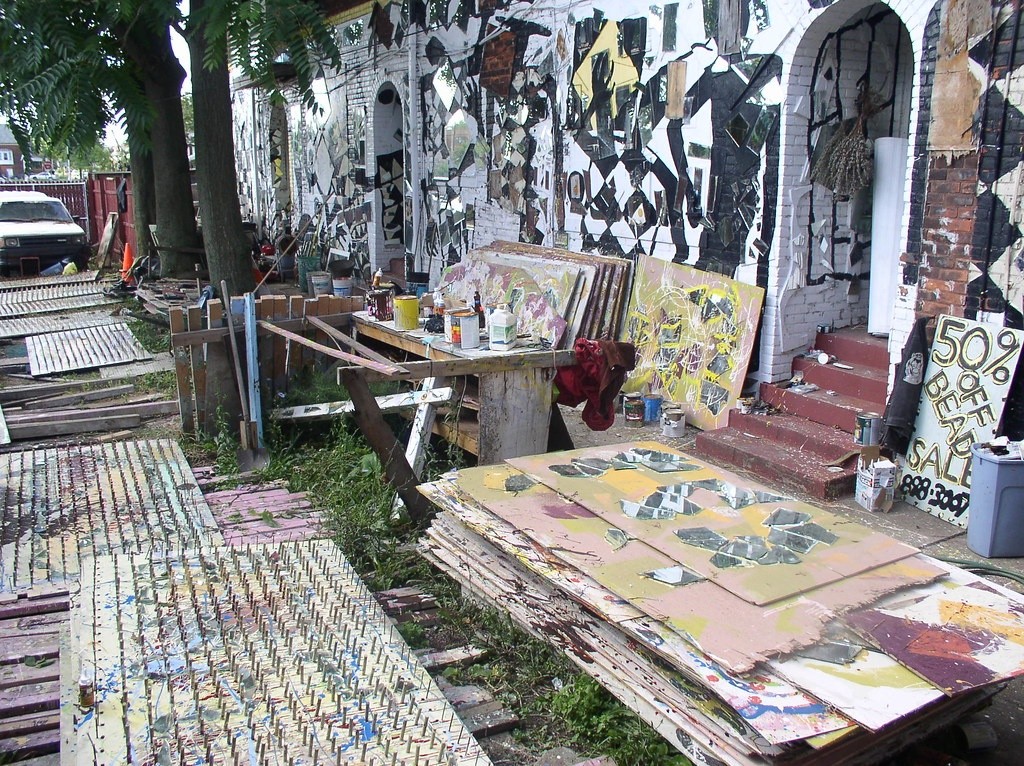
[116,244,138,285]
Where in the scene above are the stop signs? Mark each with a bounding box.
[44,161,52,171]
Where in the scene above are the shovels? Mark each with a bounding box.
[221,279,270,475]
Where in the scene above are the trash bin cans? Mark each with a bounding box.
[965,441,1024,558]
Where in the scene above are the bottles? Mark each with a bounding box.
[488,304,517,351]
[474,291,480,307]
[433,287,446,316]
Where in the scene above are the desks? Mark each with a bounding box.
[353,309,555,466]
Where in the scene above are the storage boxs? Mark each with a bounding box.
[856,445,899,515]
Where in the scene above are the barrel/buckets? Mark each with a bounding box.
[393,295,419,330]
[643,394,663,422]
[423,305,434,318]
[312,276,332,297]
[368,289,394,322]
[333,277,352,297]
[451,313,480,349]
[625,400,645,428]
[622,392,641,415]
[444,307,472,343]
[660,402,681,431]
[306,272,325,299]
[737,396,757,415]
[663,409,685,437]
[298,256,321,293]
[853,411,880,447]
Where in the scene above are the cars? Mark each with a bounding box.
[72,169,88,179]
[0,190,88,271]
[11,171,34,179]
[29,171,57,180]
